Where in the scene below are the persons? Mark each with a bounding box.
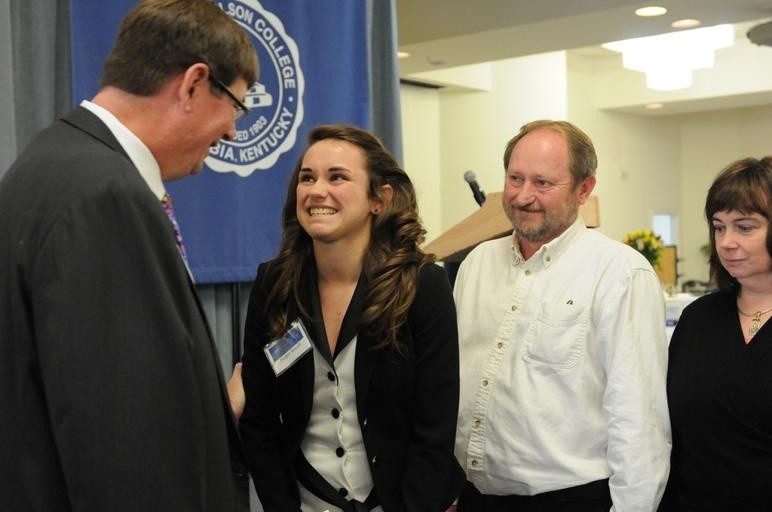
[235,125,466,512]
[656,156,772,512]
[1,1,260,512]
[453,120,673,512]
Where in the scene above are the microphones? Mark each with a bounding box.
[465,171,484,204]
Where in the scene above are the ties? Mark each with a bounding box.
[160,192,189,267]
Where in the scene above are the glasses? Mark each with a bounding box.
[185,60,252,120]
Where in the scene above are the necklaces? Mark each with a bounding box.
[738,308,772,337]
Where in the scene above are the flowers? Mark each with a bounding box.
[624,228,664,267]
